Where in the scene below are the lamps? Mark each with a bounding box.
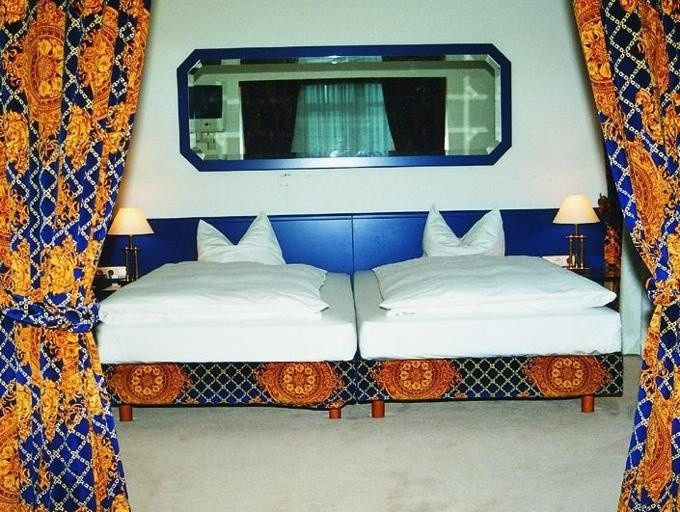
[107,205,154,284]
[552,194,602,270]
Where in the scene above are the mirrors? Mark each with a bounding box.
[176,42,513,172]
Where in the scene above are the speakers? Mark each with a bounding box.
[189,85,223,119]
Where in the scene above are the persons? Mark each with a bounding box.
[330,138,352,158]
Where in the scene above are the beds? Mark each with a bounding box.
[96,259,357,418]
[351,260,621,421]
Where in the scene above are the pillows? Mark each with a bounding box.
[420,204,506,259]
[196,212,288,266]
